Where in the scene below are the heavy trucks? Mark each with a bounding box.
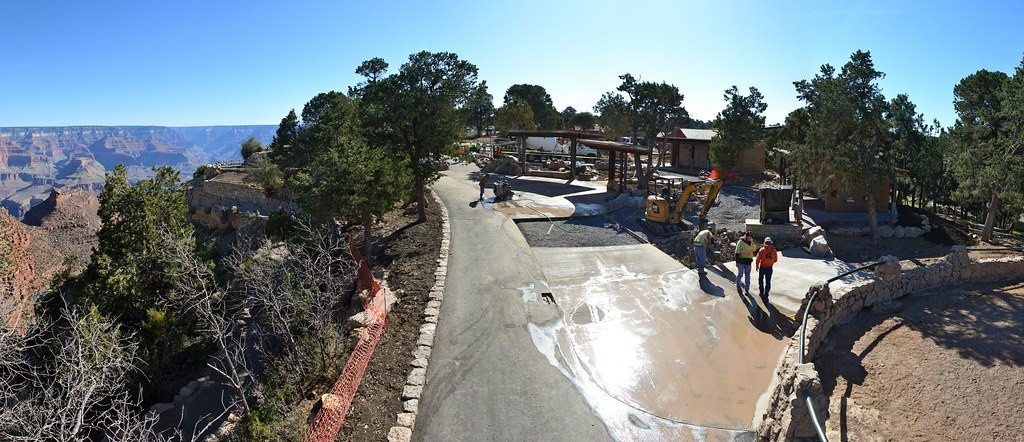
[526,136,597,157]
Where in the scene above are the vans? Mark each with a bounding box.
[620,137,640,146]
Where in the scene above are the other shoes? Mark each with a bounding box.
[698,272,707,275]
[759,293,763,298]
[745,290,749,296]
[738,287,742,292]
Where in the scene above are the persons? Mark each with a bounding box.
[756,237,777,303]
[453,141,517,158]
[693,226,715,276]
[735,231,763,299]
[560,147,564,153]
[479,173,490,201]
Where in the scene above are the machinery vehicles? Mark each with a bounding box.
[644,181,725,228]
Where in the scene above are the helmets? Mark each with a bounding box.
[765,237,773,244]
